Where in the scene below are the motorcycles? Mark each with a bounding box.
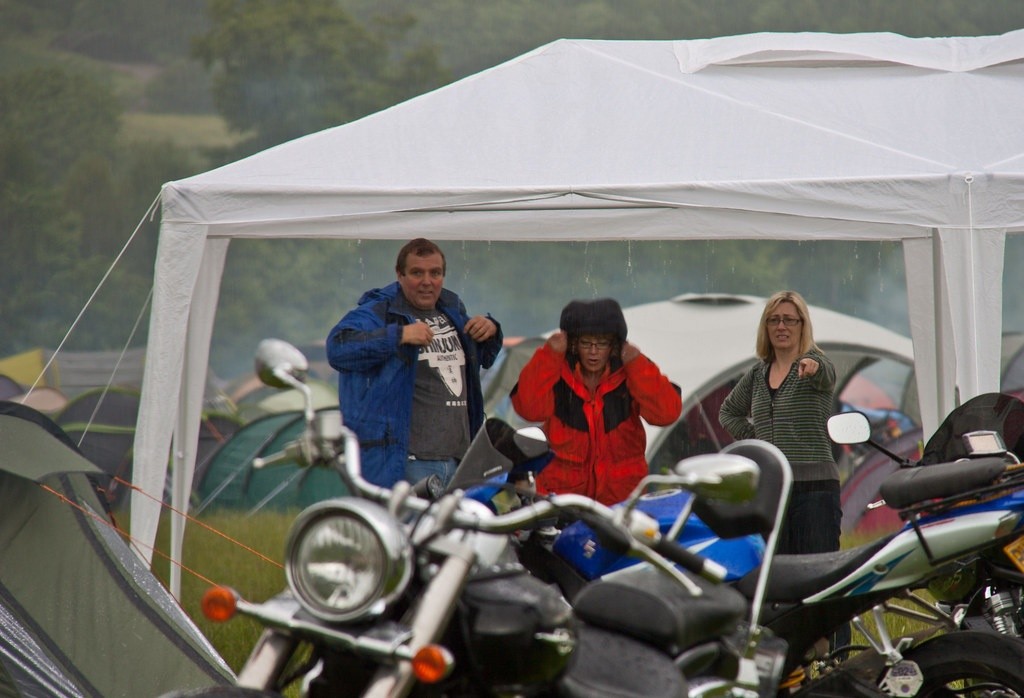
[155,335,1024,698]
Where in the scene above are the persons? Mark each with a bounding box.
[326,237,503,495]
[508,297,683,508]
[717,288,856,663]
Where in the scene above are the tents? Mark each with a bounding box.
[129,25,1023,600]
[0,348,246,698]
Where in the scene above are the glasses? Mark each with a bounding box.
[766,317,802,326]
[575,340,613,350]
